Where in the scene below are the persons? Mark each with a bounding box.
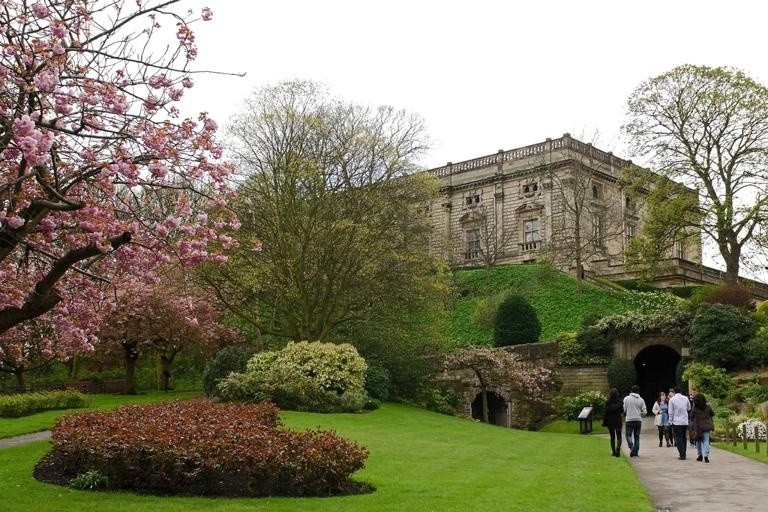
[621,385,648,458]
[651,387,715,464]
[601,387,626,457]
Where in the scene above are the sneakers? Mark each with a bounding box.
[659,443,710,463]
[612,448,640,458]
